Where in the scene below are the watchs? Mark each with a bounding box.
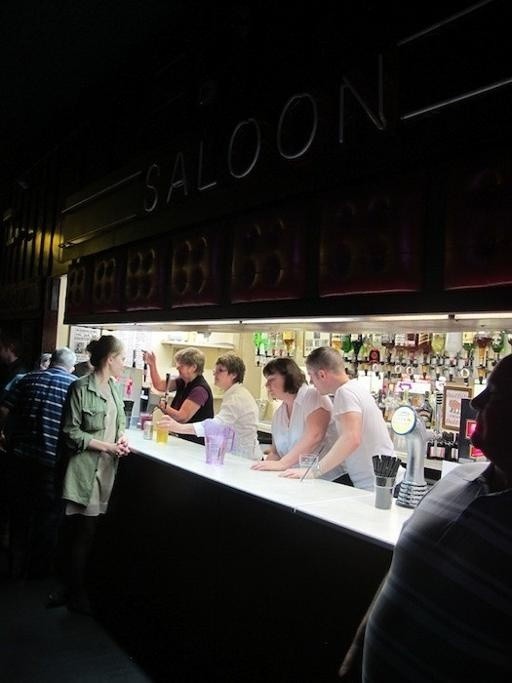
[313,462,322,477]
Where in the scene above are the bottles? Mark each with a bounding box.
[428,431,460,462]
[252,328,297,368]
[332,333,512,385]
[384,389,409,422]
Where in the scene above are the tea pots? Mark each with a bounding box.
[202,419,235,466]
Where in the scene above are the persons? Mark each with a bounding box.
[50,334,131,616]
[336,353,511,681]
[280,347,405,498]
[1,346,80,572]
[250,357,354,487]
[157,355,265,460]
[2,353,53,569]
[141,347,214,445]
[0,337,35,560]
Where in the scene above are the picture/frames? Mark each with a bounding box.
[443,385,471,431]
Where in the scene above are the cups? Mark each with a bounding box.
[373,475,395,510]
[156,421,170,445]
[299,455,319,488]
[411,396,421,407]
